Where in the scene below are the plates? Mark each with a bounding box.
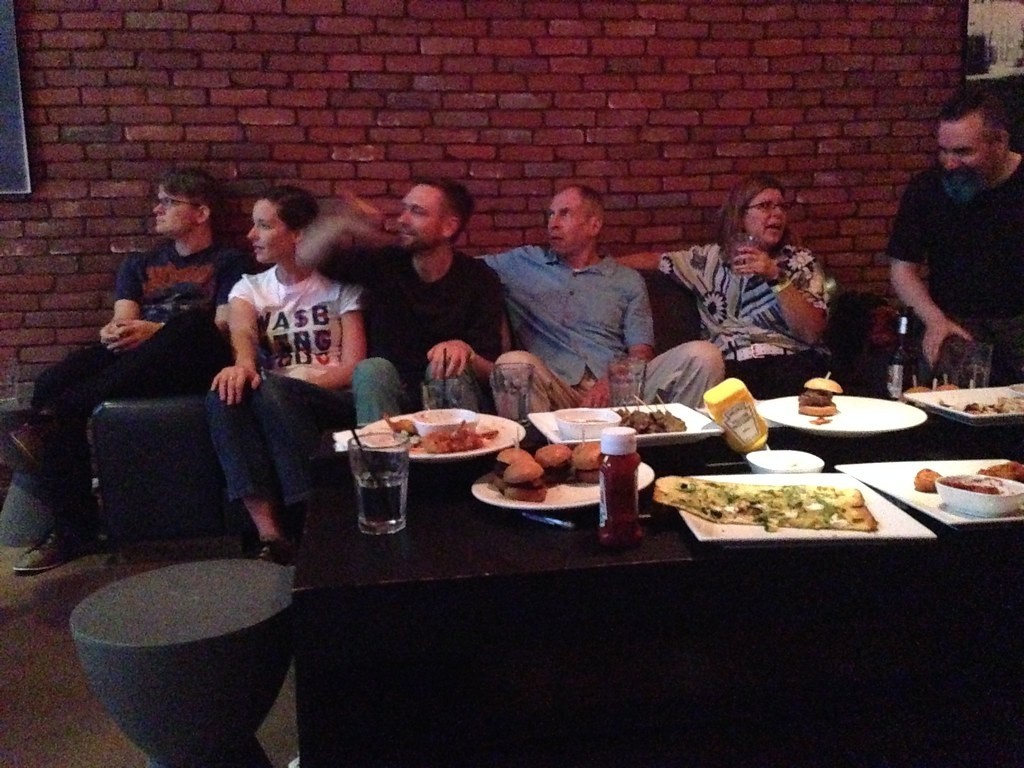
[357,413,525,463]
[757,395,928,438]
[674,472,937,549]
[835,458,1024,526]
[527,402,725,446]
[746,450,824,475]
[902,387,1024,426]
[471,461,655,511]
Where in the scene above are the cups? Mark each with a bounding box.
[933,340,992,389]
[489,363,535,428]
[420,380,463,411]
[730,233,764,275]
[348,433,409,535]
[608,358,646,406]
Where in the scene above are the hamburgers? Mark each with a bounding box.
[798,377,844,416]
[492,442,603,504]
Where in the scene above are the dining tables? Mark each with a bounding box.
[293,408,1024,767]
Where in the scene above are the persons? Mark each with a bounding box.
[886,88,1024,388]
[204,185,367,568]
[617,174,833,400]
[338,175,512,428]
[474,182,726,421]
[7,161,250,570]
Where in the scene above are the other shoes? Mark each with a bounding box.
[249,539,293,567]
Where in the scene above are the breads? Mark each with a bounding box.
[904,383,958,393]
[976,462,1024,485]
[914,468,944,493]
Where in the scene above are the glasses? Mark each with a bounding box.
[154,196,202,208]
[744,201,792,213]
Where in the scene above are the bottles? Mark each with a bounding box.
[703,377,771,462]
[597,427,644,548]
[885,305,918,400]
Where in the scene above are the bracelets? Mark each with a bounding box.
[766,271,792,294]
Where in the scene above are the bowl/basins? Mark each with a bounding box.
[552,407,622,439]
[413,409,477,438]
[935,473,1023,518]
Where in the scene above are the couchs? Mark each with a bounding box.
[85,267,890,541]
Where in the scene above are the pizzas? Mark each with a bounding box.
[651,475,879,533]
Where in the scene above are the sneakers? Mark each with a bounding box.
[13,523,98,571]
[9,422,47,463]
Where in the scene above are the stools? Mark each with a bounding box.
[69,557,295,768]
[0,398,93,548]
